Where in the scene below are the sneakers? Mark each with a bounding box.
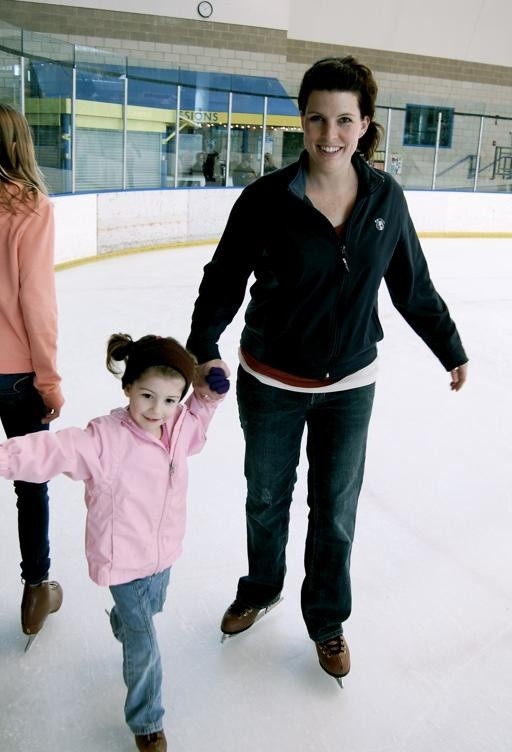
[21,580,62,635]
[221,600,256,635]
[316,634,350,677]
[135,730,167,752]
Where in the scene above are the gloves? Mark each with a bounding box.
[204,366,230,395]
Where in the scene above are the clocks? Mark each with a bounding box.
[197,1,213,18]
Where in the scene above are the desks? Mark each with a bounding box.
[167,175,205,187]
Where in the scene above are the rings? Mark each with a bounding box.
[202,393,208,400]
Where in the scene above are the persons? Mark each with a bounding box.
[0,329,230,752]
[192,52,472,696]
[1,105,68,656]
[187,148,278,188]
